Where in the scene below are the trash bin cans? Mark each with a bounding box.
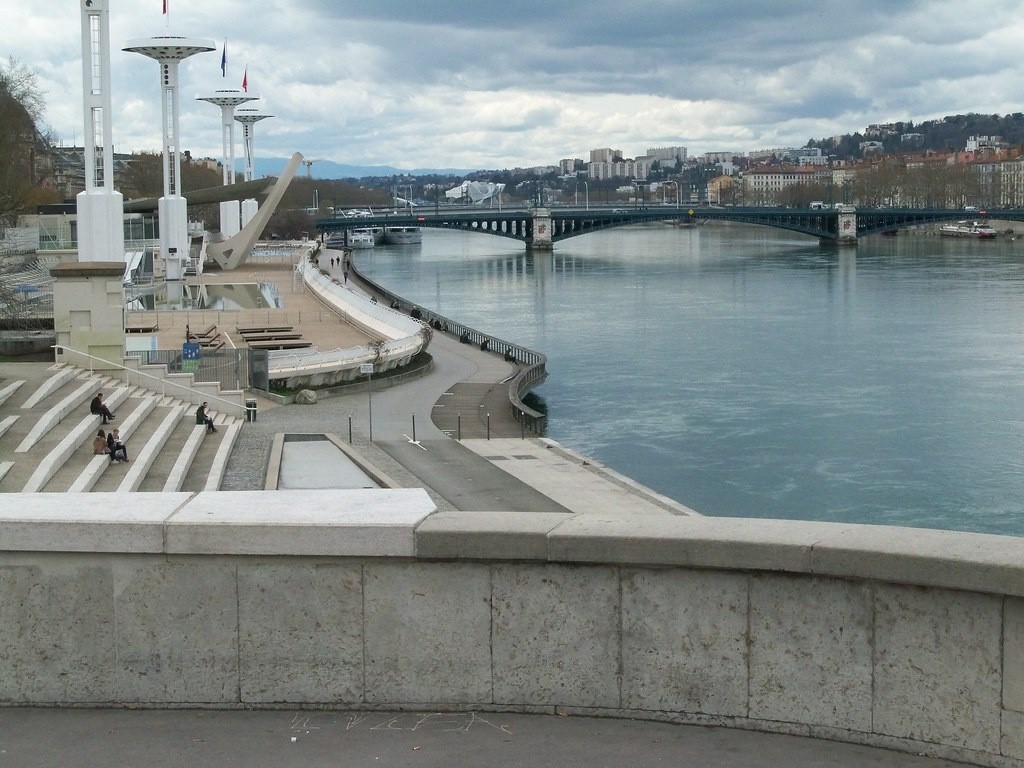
[245,398,257,422]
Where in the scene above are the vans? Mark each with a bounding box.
[965,205,980,214]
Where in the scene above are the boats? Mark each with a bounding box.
[295,188,424,252]
[939,220,998,239]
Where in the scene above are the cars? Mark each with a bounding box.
[875,204,887,211]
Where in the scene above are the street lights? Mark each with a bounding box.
[575,182,579,205]
[498,187,502,213]
[490,188,492,207]
[674,182,682,210]
[584,182,588,211]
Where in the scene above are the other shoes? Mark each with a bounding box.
[111,459,119,465]
[124,458,129,462]
[212,426,218,432]
[103,422,109,424]
[207,429,214,434]
[109,417,115,420]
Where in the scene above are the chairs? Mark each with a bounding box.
[199,330,221,348]
[242,331,303,341]
[247,340,312,350]
[202,341,227,357]
[189,323,217,339]
[236,324,293,334]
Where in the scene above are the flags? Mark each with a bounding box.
[242,70,248,92]
[163,0,166,14]
[221,43,226,77]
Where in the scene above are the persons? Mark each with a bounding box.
[316,259,319,264]
[331,258,334,268]
[93,429,129,464]
[344,272,348,284]
[371,296,377,302]
[196,402,217,434]
[429,318,446,332]
[342,251,350,270]
[460,332,471,344]
[391,300,399,310]
[505,348,517,365]
[336,256,340,266]
[90,393,116,424]
[410,307,422,320]
[481,340,490,351]
